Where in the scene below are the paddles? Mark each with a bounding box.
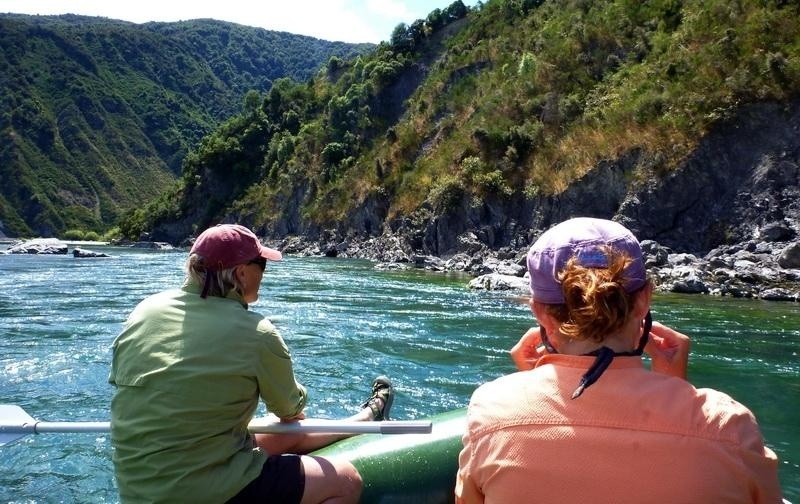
[0,405,432,448]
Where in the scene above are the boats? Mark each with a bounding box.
[305,406,469,504]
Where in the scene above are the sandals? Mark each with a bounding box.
[367,376,393,422]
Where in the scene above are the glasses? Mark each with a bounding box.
[250,257,267,272]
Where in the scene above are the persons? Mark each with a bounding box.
[451,212,787,503]
[104,220,396,503]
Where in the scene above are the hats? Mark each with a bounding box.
[188,223,283,272]
[527,217,647,305]
